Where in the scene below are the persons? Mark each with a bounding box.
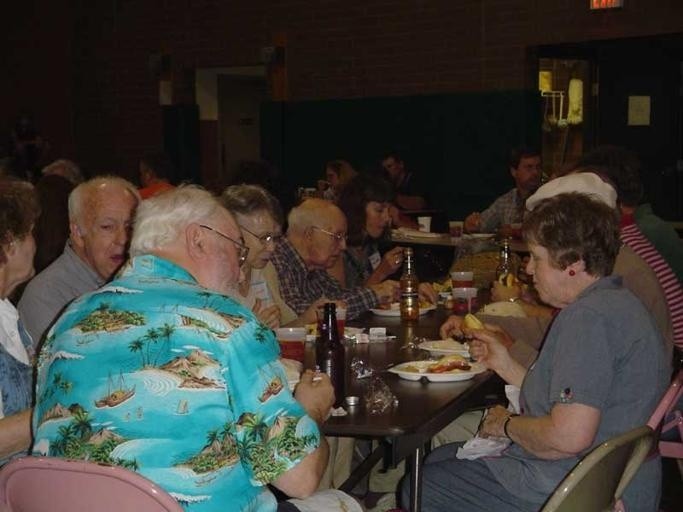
[137,151,179,198]
[317,159,357,200]
[614,169,683,290]
[268,198,398,320]
[0,177,38,469]
[466,150,550,240]
[569,166,683,379]
[17,178,141,351]
[429,173,673,451]
[399,192,667,512]
[327,185,403,294]
[32,188,367,511]
[220,183,339,338]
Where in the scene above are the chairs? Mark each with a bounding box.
[0,457,184,512]
[616,370,683,512]
[541,424,654,512]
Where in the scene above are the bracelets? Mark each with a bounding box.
[503,413,520,439]
[509,296,520,302]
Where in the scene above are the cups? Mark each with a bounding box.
[418,217,431,233]
[277,328,305,364]
[317,309,346,337]
[451,271,474,288]
[450,221,463,242]
[453,287,478,318]
[511,223,523,240]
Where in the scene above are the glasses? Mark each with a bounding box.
[313,226,349,243]
[200,224,250,268]
[239,223,283,247]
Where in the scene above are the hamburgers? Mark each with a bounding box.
[498,272,519,287]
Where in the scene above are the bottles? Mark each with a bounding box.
[496,242,515,280]
[400,248,420,320]
[318,303,345,406]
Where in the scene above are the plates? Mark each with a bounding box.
[386,360,487,381]
[368,303,438,316]
[417,340,474,359]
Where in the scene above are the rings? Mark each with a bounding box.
[395,260,399,264]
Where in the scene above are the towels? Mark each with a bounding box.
[455,384,521,461]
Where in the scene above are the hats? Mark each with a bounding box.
[525,170,617,209]
